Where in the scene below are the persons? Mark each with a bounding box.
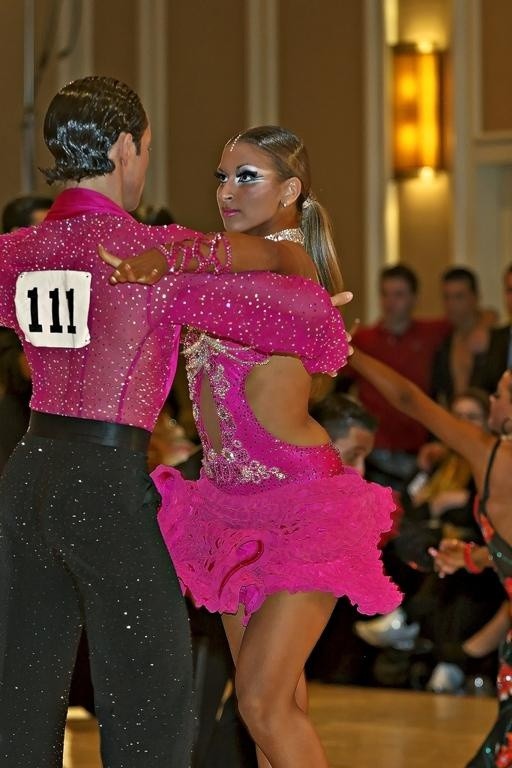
[336,318,511,768]
[96,124,401,768]
[2,72,353,768]
[2,193,257,768]
[299,261,512,693]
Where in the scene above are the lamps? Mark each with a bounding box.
[386,38,443,183]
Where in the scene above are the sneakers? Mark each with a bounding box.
[427,664,466,692]
[356,610,421,653]
